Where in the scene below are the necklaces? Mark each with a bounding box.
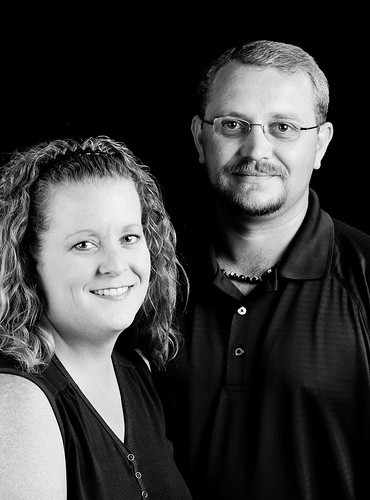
[220,268,270,284]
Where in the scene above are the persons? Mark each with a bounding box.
[0,135,190,500]
[151,41,370,500]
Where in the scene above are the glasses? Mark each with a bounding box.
[202,116,319,143]
